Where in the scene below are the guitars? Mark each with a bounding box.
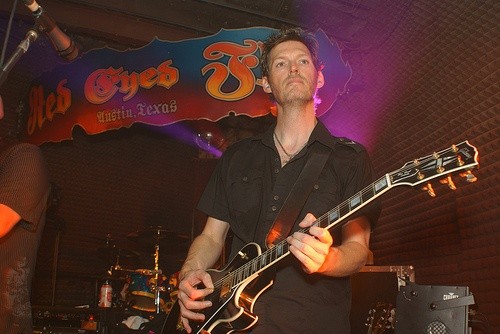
[160,137,480,334]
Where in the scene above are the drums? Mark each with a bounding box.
[128,267,162,311]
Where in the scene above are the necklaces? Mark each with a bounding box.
[273,132,304,165]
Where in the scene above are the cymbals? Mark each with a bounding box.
[135,223,188,243]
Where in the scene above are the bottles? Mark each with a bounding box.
[98,280,113,310]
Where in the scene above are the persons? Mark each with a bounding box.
[177,26,382,334]
[0,95,50,334]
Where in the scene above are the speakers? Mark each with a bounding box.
[394,284,475,334]
